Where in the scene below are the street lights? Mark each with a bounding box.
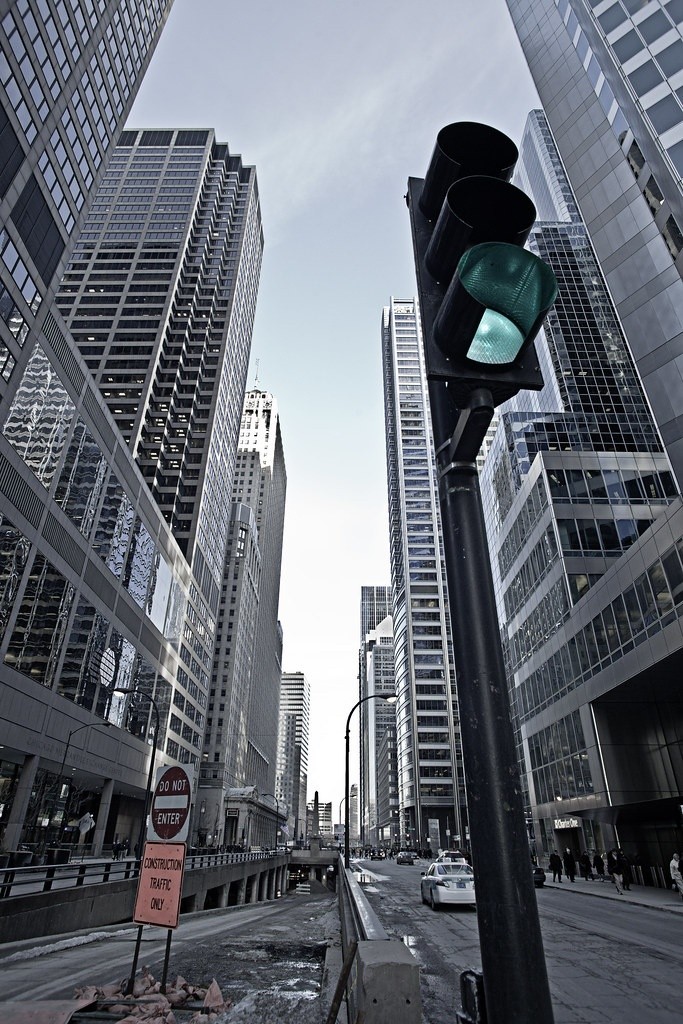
[38,722,110,868]
[299,818,308,847]
[339,794,357,852]
[223,792,245,850]
[345,692,399,868]
[197,799,206,843]
[213,802,220,847]
[394,809,409,851]
[110,686,161,877]
[261,793,279,851]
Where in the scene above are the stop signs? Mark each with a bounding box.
[149,763,195,845]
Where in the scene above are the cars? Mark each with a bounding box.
[532,860,546,888]
[371,848,385,860]
[398,851,414,865]
[421,862,476,910]
[410,852,419,860]
[436,851,469,865]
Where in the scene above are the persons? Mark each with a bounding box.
[351,846,434,860]
[112,839,129,861]
[217,842,245,854]
[548,848,634,896]
[670,854,683,898]
[134,842,139,858]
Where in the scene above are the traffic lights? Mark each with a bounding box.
[408,122,560,388]
